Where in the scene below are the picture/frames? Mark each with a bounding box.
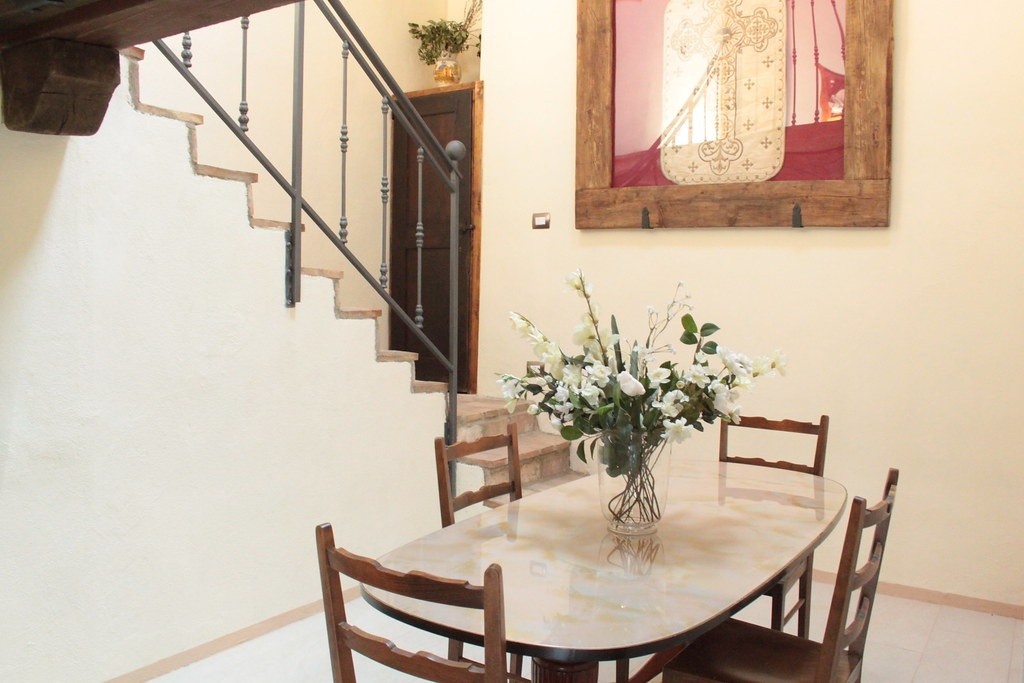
[575,0,893,229]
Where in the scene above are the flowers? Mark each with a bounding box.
[494,265,793,528]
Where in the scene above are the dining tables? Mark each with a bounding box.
[359,459,847,683]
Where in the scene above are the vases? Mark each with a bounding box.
[596,429,674,536]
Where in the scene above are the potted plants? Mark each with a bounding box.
[408,0,483,87]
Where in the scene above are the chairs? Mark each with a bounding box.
[718,412,830,640]
[315,522,507,683]
[662,467,899,683]
[434,421,526,681]
